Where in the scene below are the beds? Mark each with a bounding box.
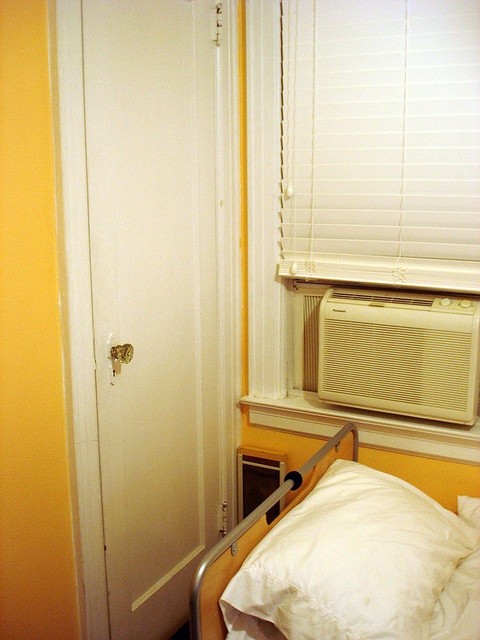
[189,419,479,640]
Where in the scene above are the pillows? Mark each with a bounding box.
[218,457,479,640]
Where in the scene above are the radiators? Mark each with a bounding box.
[284,277,480,429]
